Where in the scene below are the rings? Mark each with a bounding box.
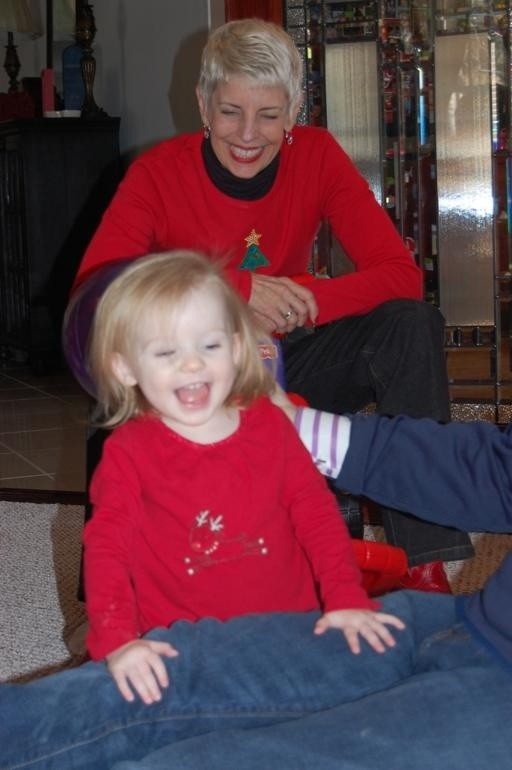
[284,311,294,319]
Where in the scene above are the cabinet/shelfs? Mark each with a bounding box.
[0,115,121,378]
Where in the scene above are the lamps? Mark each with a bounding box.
[0,0,41,122]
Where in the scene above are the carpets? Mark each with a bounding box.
[1,486,483,687]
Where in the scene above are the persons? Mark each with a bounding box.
[66,18,487,612]
[67,243,407,706]
[0,375,512,768]
